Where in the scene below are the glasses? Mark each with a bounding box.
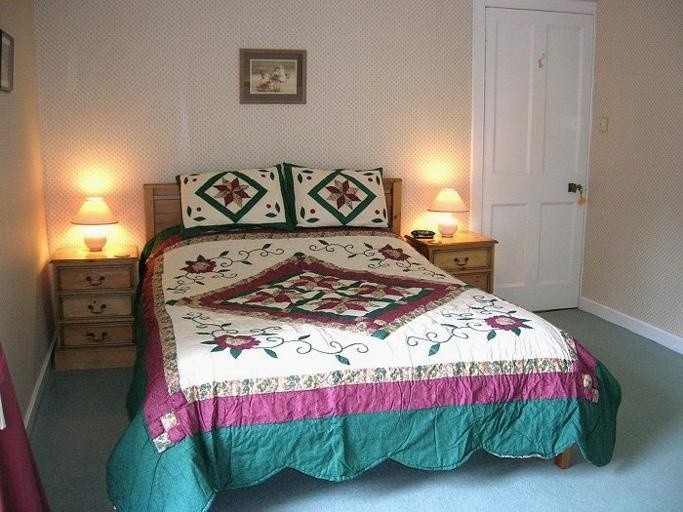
[427,188,467,238]
[70,197,116,251]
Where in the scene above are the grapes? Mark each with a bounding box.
[405,232,499,292]
[46,247,143,373]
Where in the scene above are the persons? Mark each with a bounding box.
[259,72,270,85]
[271,65,280,80]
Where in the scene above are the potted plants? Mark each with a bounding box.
[1,30,15,91]
[238,47,306,105]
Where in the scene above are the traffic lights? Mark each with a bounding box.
[179,163,391,234]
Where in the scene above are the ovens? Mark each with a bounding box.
[143,179,572,510]
